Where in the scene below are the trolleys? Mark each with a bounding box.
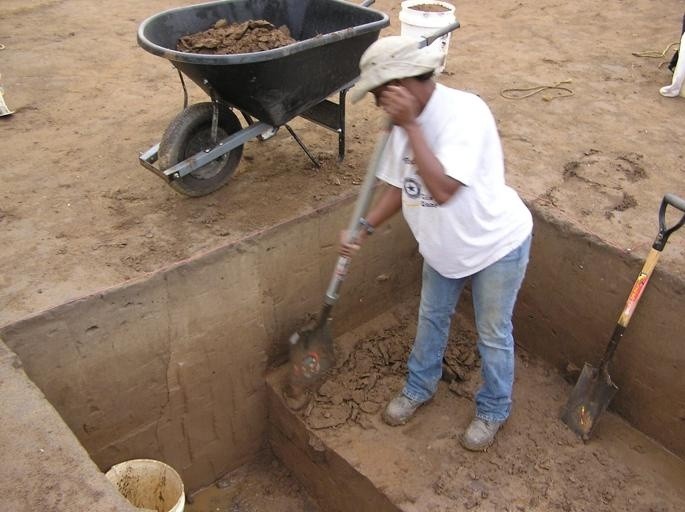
[131,0,463,200]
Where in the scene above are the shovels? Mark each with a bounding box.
[287,95,395,393]
[559,193,685,441]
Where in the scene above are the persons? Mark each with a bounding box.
[336,36,534,451]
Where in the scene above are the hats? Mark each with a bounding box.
[350,36,446,103]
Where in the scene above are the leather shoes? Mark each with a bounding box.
[462,416,504,451]
[381,392,432,426]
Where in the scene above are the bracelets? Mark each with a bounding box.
[358,218,376,235]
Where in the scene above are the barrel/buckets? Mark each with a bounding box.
[105,459,186,512]
